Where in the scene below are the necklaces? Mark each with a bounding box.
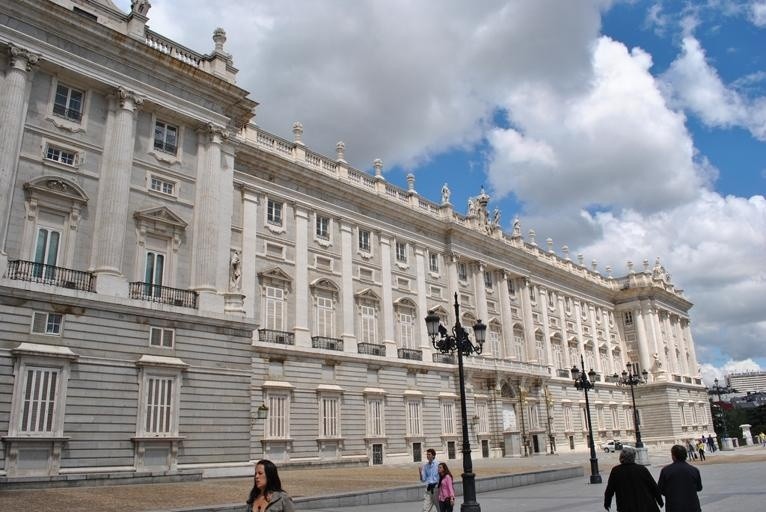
[254,491,273,511]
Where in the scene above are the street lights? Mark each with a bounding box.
[571,354,603,483]
[426,292,486,511]
[613,362,650,448]
[705,378,731,438]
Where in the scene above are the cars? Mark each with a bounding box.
[600,441,633,453]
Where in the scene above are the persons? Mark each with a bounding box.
[685,432,717,462]
[603,446,664,512]
[656,445,703,512]
[418,448,441,512]
[245,458,297,511]
[437,462,455,512]
[759,431,766,445]
